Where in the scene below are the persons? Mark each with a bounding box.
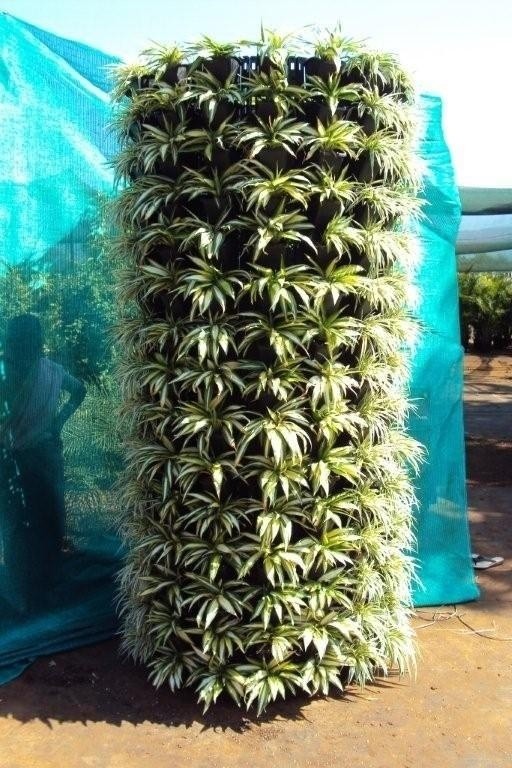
[2,312,87,613]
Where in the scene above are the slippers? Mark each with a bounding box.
[471,554,504,569]
